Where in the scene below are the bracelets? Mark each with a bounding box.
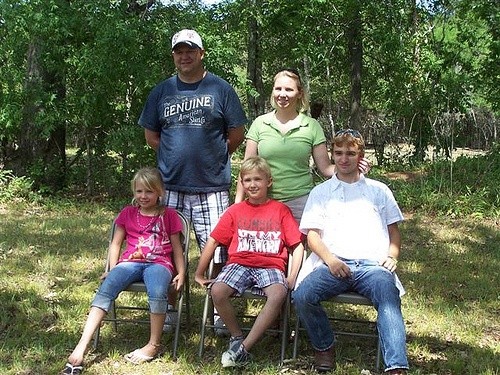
[387,256,398,262]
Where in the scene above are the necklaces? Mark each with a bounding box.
[136,207,157,228]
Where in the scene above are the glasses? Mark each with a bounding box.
[334,129,361,138]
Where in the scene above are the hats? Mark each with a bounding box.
[171,28,203,53]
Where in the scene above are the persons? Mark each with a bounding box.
[293,129,410,375]
[194,156,304,368]
[137,29,249,337]
[61,166,186,375]
[235,70,371,226]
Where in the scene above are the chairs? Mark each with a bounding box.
[94,210,190,360]
[292,290,383,371]
[198,245,290,363]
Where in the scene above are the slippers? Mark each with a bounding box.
[61,362,83,375]
[124,341,163,365]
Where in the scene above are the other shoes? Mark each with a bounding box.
[213,314,232,335]
[314,344,336,371]
[221,336,251,367]
[163,312,178,333]
